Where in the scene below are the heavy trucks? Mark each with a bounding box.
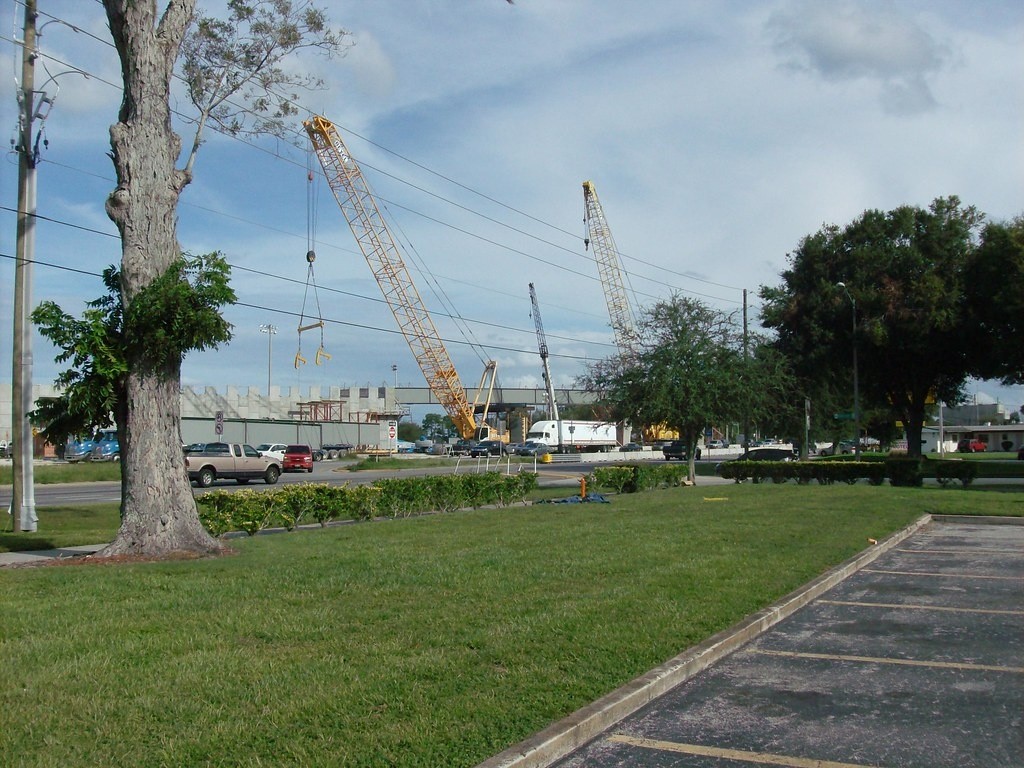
[525,420,617,453]
[63,438,95,465]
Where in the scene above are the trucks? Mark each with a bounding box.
[94,430,124,463]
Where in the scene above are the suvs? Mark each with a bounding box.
[471,440,508,459]
[957,438,987,452]
[733,449,799,462]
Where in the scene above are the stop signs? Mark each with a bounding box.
[388,426,396,440]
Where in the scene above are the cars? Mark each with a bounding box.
[257,443,314,475]
[618,442,642,453]
[751,438,775,447]
[820,442,863,457]
[505,442,551,457]
[706,440,723,449]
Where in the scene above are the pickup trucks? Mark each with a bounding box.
[183,441,284,488]
[662,441,702,460]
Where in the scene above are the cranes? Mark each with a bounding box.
[293,113,510,454]
[580,178,680,451]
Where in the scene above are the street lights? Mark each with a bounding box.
[836,281,860,463]
